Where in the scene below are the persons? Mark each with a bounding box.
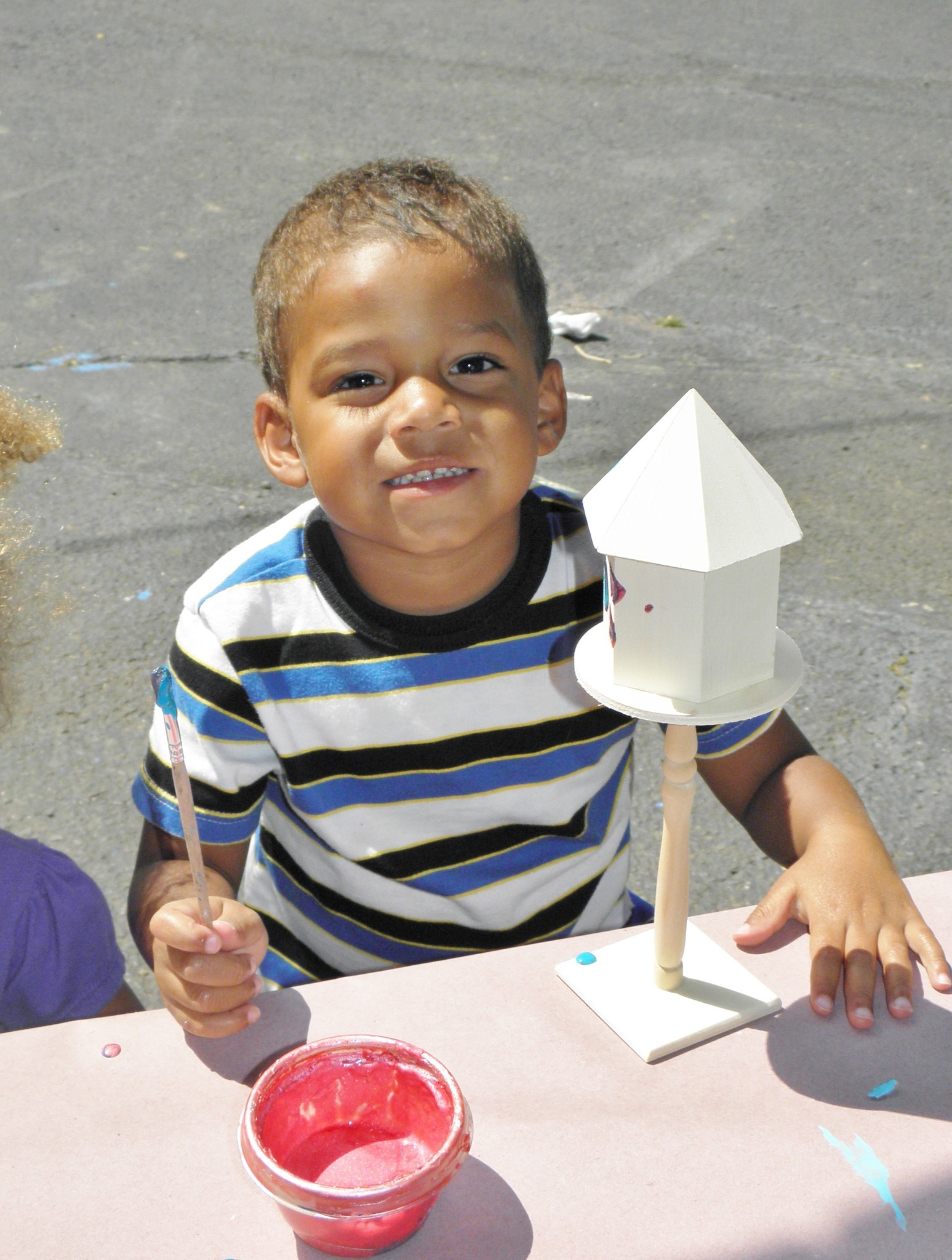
[0,828,145,1035]
[125,149,952,1034]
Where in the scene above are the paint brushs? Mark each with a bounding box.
[152,665,215,932]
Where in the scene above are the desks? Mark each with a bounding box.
[2,863,952,1259]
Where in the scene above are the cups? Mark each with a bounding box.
[238,1033,474,1257]
